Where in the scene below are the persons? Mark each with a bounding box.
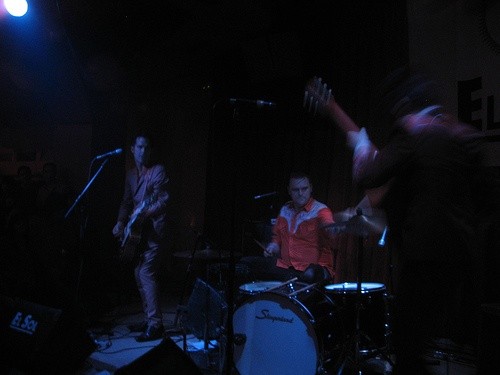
[112,134,170,344]
[263,172,339,295]
[346,71,484,375]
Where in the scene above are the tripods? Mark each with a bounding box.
[332,233,395,375]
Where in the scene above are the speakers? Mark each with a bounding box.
[183,277,233,341]
[0,297,98,375]
[116,339,203,375]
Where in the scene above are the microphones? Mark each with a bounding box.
[254,192,277,200]
[378,227,387,247]
[233,334,244,345]
[230,98,265,106]
[194,231,216,248]
[96,148,123,159]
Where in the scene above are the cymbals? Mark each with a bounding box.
[172,245,231,260]
[321,208,389,236]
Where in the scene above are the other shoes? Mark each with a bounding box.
[137,327,162,340]
[128,321,149,332]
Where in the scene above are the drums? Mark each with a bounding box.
[239,280,285,297]
[226,282,342,375]
[325,282,388,351]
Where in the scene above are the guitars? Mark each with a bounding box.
[119,189,159,262]
[302,74,394,206]
